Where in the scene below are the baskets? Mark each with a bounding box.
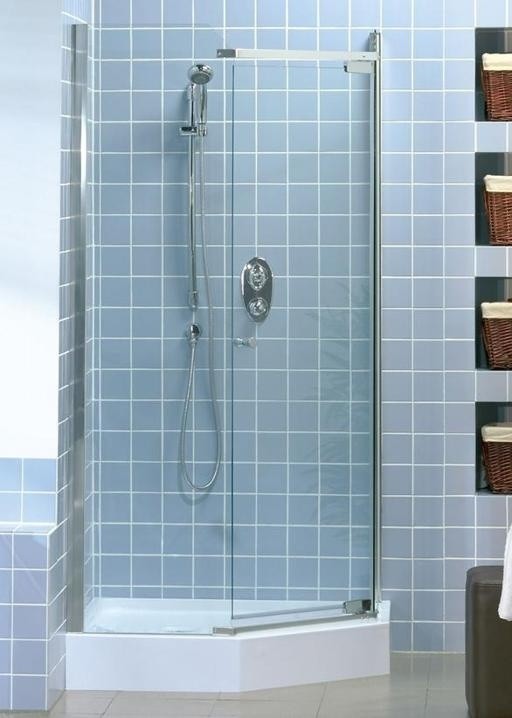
[480,68,511,121]
[481,440,512,495]
[483,188,512,248]
[480,316,512,371]
